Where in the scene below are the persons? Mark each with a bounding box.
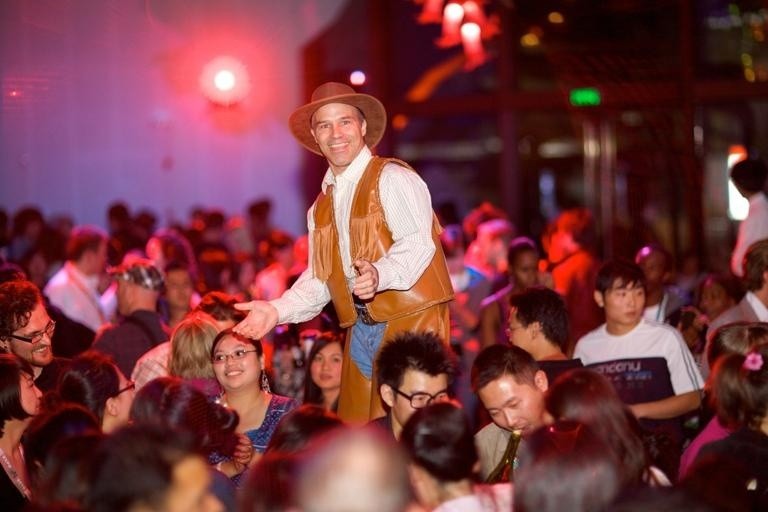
[231,84,454,424]
[0,195,767,512]
[726,155,768,280]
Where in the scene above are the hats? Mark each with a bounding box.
[289,82,388,156]
[106,260,165,292]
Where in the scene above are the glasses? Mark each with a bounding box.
[213,349,260,364]
[11,319,56,343]
[399,387,448,411]
[117,380,136,395]
